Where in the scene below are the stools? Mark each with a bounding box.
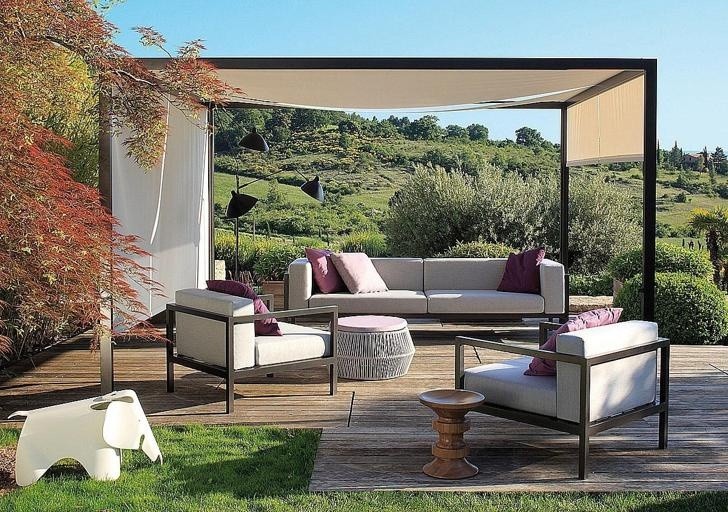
[418,388,486,480]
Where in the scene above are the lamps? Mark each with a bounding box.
[226,127,324,281]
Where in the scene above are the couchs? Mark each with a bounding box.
[283,257,565,321]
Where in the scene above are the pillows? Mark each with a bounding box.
[523,307,624,377]
[204,279,283,337]
[497,246,545,295]
[304,246,387,294]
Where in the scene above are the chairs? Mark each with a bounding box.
[453,320,669,479]
[166,288,338,413]
